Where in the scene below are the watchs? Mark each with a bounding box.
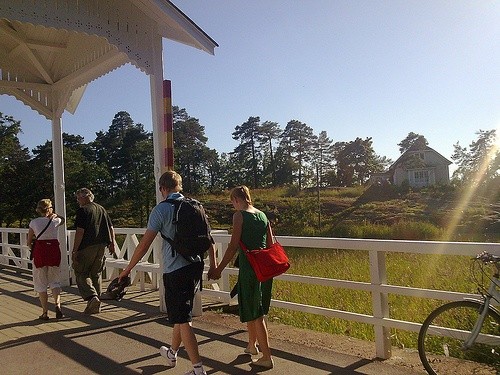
[72,251,76,254]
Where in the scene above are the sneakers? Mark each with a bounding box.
[159,346,177,367]
[184,368,207,375]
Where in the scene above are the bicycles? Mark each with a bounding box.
[418,249,500,375]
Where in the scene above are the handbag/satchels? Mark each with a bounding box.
[102,205,113,243]
[30,240,34,260]
[244,242,290,282]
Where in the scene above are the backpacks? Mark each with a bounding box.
[159,196,216,291]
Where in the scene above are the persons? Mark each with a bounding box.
[117,170,221,375]
[28,199,66,320]
[207,185,274,368]
[72,188,115,315]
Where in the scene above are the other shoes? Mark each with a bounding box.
[244,347,259,355]
[84,297,101,313]
[56,309,63,318]
[39,310,49,320]
[252,358,273,368]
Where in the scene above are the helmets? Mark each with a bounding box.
[106,275,131,300]
